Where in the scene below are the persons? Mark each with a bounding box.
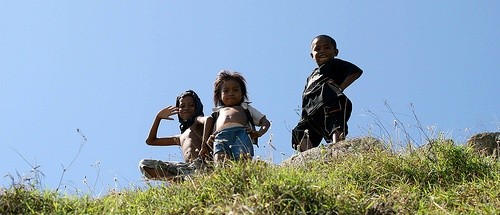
[291,34,363,152]
[206,69,271,170]
[139,89,214,185]
